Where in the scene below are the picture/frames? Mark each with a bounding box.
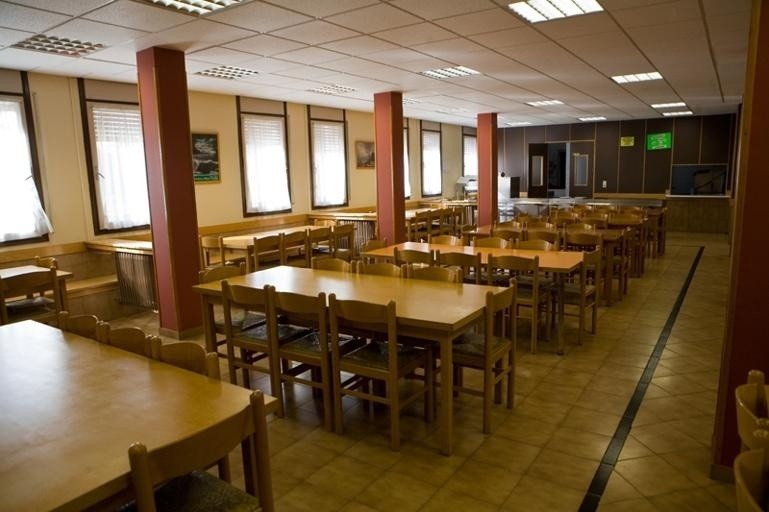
[190,130,220,184]
[354,142,375,169]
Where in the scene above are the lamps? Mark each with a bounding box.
[418,65,480,80]
[608,71,663,84]
[12,34,106,59]
[195,66,258,81]
[510,1,603,26]
[135,0,252,20]
[523,99,564,107]
[307,85,354,97]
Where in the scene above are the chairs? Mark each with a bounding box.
[452,278,518,430]
[220,280,433,451]
[36,256,61,314]
[0,266,63,324]
[393,247,552,355]
[414,203,470,239]
[460,203,667,299]
[50,310,234,483]
[563,244,603,346]
[128,389,275,512]
[731,370,768,511]
[361,237,390,266]
[277,255,464,398]
[199,218,356,275]
[427,233,561,339]
[187,263,268,390]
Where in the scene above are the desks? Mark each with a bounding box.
[359,240,583,355]
[192,264,511,456]
[463,224,624,307]
[1,264,73,322]
[404,208,462,242]
[0,319,279,512]
[219,226,338,273]
